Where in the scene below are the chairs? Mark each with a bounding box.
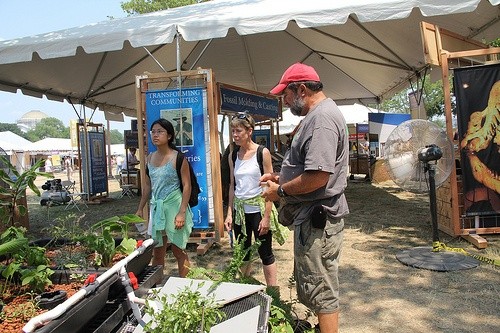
[114,175,136,200]
[61,180,89,211]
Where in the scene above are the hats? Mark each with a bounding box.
[268,62,321,94]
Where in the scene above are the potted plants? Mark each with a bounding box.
[0,148,158,333]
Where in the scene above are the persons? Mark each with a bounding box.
[223,112,276,287]
[135,118,194,278]
[258,62,349,333]
[44,148,140,173]
[351,135,369,153]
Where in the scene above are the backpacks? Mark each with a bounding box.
[145,151,201,208]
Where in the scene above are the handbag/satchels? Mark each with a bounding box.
[311,206,327,228]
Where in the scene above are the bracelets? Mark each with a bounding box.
[277,184,287,197]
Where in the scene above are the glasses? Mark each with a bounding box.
[229,114,254,129]
[150,128,167,135]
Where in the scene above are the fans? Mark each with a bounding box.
[383,119,480,271]
